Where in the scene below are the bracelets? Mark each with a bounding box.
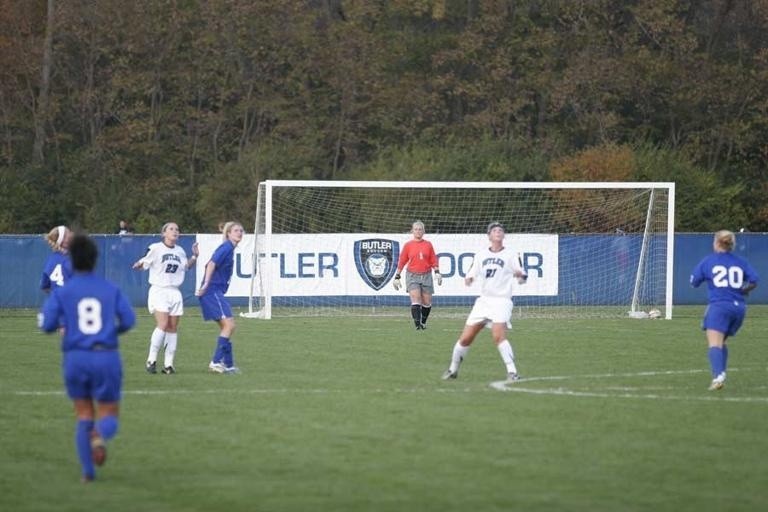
[191,253,199,260]
[434,269,440,274]
[394,273,402,280]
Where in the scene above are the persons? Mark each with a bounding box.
[128,220,199,377]
[115,219,133,233]
[392,217,441,334]
[37,223,74,296]
[686,228,758,394]
[35,232,137,486]
[441,219,528,381]
[193,219,245,377]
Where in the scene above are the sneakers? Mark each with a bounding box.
[440,370,459,381]
[161,366,176,376]
[79,428,109,483]
[704,371,729,393]
[414,323,427,332]
[506,372,522,381]
[208,360,237,374]
[145,362,158,375]
